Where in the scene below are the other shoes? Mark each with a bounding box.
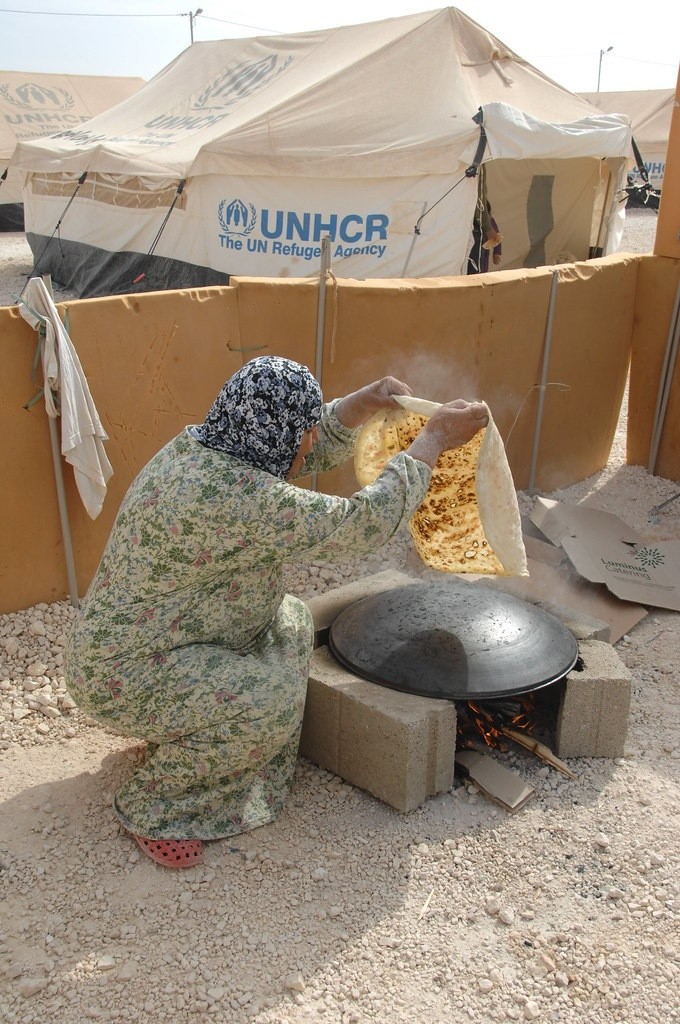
[136,833,202,868]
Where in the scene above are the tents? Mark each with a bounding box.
[0,69,147,231]
[573,88,676,207]
[9,6,632,300]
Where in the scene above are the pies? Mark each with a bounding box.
[353,394,530,578]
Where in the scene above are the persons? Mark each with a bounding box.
[467,200,501,274]
[64,355,489,868]
